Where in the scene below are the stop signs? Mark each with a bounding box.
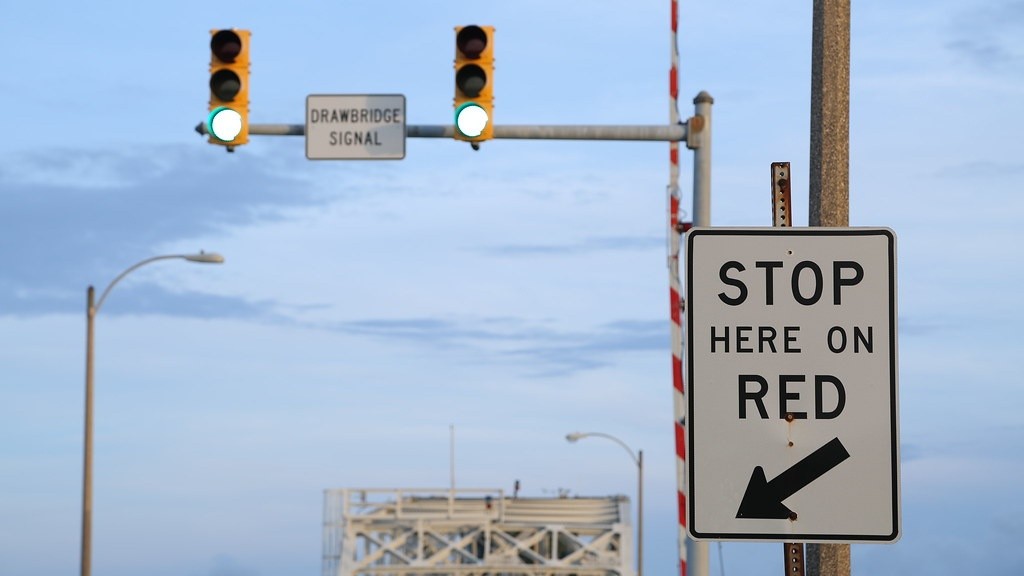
[684,224,903,544]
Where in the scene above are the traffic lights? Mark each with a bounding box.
[451,23,495,146]
[208,27,252,149]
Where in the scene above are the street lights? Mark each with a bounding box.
[76,250,226,576]
[565,432,644,576]
